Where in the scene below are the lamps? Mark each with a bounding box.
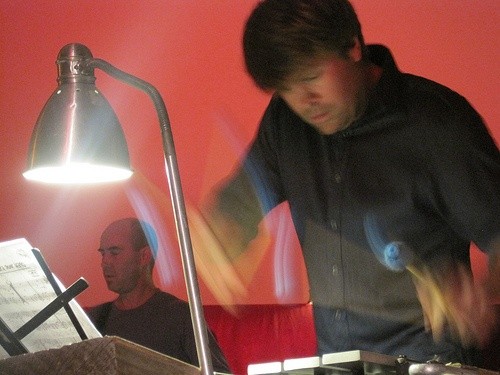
[21,43,216,374]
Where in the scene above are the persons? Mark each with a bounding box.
[130,0,500,375]
[86,217,232,374]
